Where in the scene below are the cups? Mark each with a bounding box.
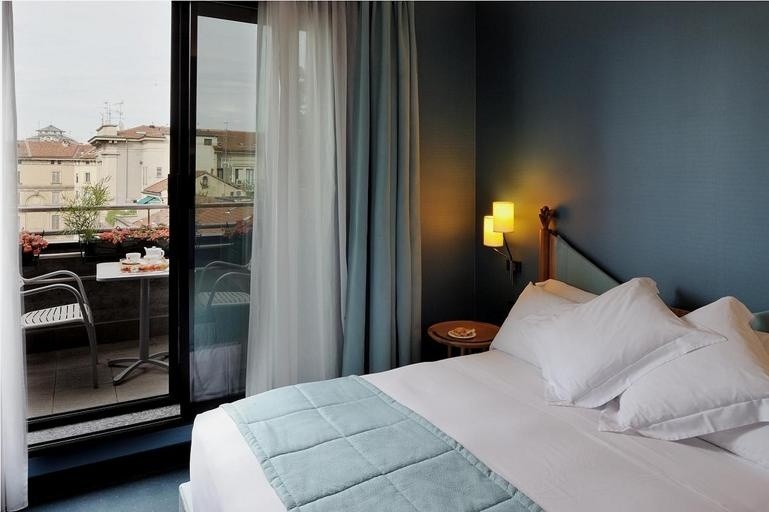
[120,252,141,272]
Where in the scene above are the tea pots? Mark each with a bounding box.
[144,246,165,258]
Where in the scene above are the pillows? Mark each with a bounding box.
[489,281,577,368]
[698,330,769,468]
[536,279,598,304]
[599,296,769,442]
[519,277,727,409]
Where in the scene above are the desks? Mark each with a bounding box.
[428,320,500,358]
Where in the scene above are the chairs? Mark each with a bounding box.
[194,257,250,388]
[20,270,99,389]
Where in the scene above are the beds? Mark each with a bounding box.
[179,206,769,512]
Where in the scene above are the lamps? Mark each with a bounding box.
[483,201,521,285]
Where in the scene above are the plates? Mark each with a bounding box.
[448,329,476,339]
